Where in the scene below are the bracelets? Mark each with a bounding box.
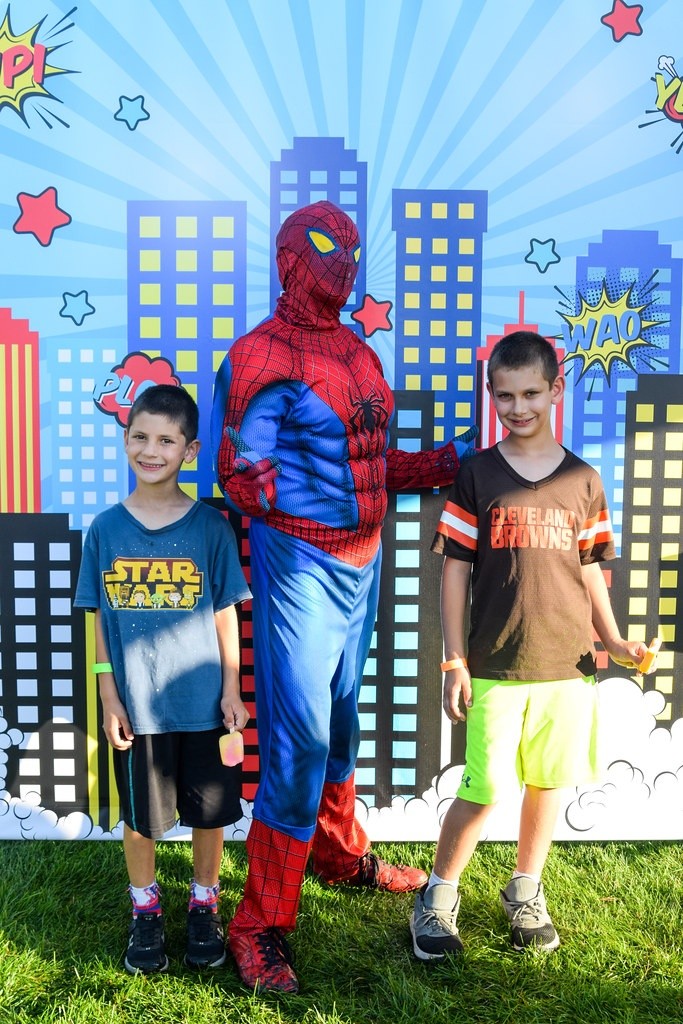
[440,658,467,672]
[92,663,113,673]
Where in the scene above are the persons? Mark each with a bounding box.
[212,201,464,995]
[407,330,657,963]
[73,385,252,974]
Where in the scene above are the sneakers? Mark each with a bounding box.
[410,882,465,959]
[125,911,169,974]
[183,906,227,970]
[234,926,299,995]
[499,877,562,950]
[343,852,429,892]
